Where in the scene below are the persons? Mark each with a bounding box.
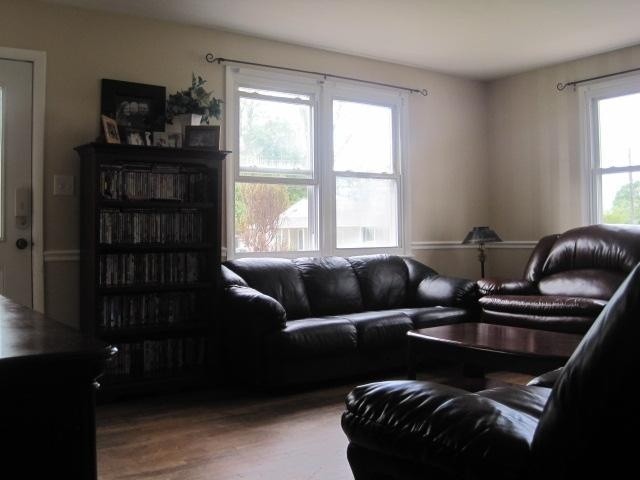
[114,101,132,128]
[129,101,141,128]
[139,102,164,131]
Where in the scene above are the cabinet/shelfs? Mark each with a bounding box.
[75,147,231,402]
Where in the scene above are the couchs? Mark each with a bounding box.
[221,257,480,402]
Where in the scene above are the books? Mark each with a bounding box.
[98,167,214,377]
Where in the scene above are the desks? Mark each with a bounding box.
[0,293,117,480]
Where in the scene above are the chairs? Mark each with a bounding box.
[340,262,640,480]
[476,226,640,335]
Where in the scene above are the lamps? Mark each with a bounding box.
[462,227,503,280]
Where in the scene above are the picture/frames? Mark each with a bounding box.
[101,115,122,144]
[184,125,220,151]
[101,79,166,143]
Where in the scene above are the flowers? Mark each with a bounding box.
[167,72,223,123]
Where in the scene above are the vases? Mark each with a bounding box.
[179,112,202,148]
